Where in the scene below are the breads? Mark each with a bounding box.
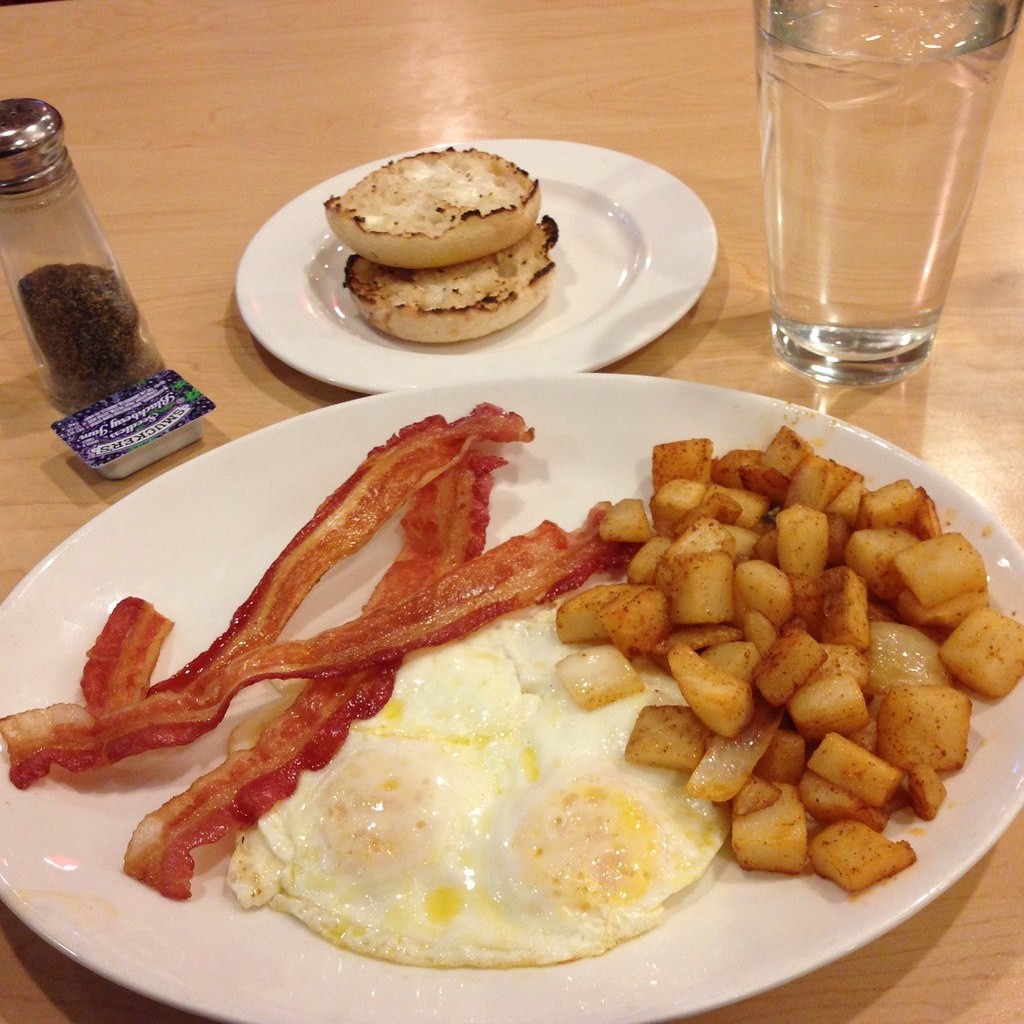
[322,145,560,344]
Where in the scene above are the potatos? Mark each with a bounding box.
[556,427,1024,891]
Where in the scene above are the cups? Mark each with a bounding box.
[754,1,1024,386]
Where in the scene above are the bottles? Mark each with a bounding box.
[0,97,167,415]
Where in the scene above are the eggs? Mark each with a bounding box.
[227,600,733,970]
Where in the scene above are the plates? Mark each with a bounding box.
[236,139,719,394]
[0,371,1024,1024]
